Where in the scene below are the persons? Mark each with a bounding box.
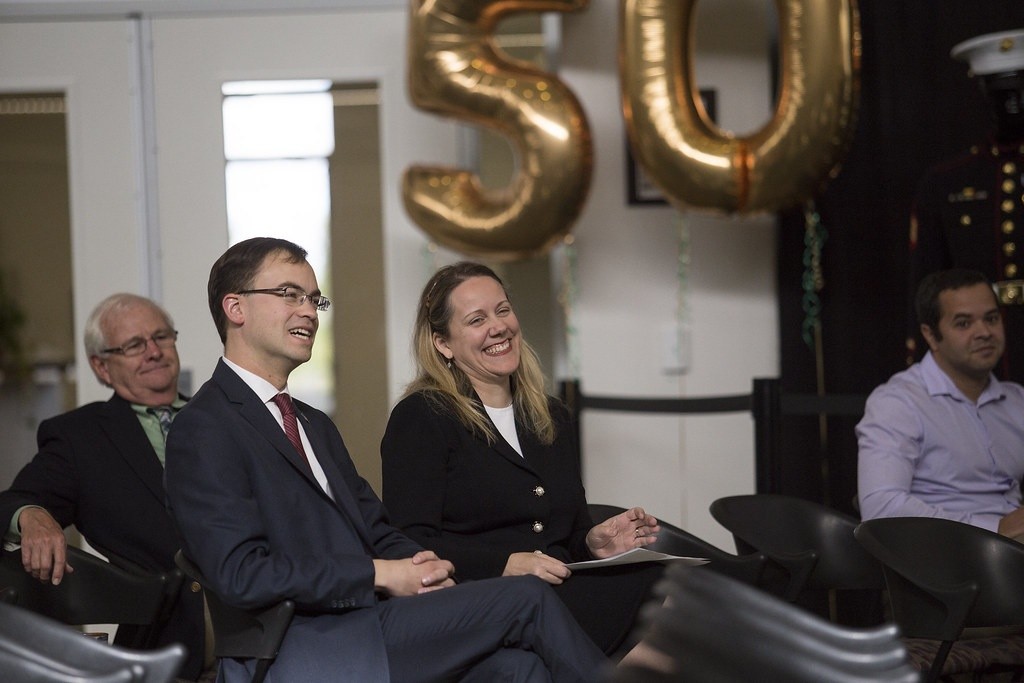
[380,262,772,660]
[854,268,1024,624]
[0,294,218,683]
[164,236,614,683]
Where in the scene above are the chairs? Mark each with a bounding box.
[0,493,1024,683]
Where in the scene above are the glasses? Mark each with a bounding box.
[233,287,330,311]
[100,330,178,357]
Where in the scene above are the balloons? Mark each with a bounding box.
[399,0,593,258]
[617,0,861,218]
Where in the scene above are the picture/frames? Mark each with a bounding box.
[628,90,717,205]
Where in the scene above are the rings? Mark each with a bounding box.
[636,528,640,537]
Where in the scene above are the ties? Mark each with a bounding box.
[152,409,172,445]
[268,393,312,475]
